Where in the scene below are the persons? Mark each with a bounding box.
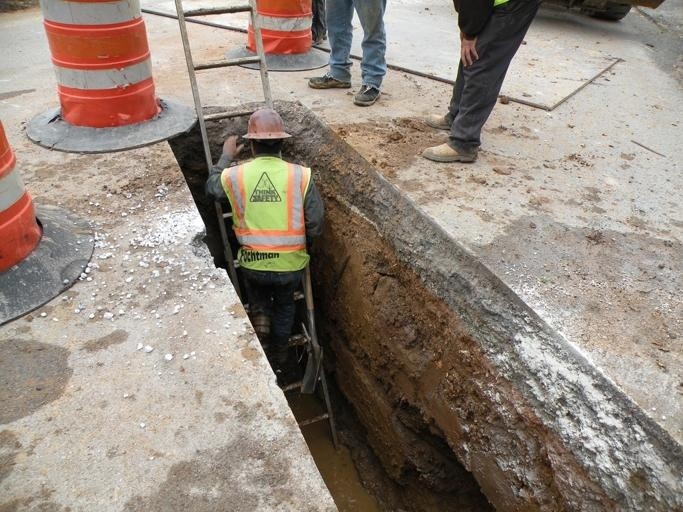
[308,0,387,106]
[311,0,327,47]
[206,108,325,364]
[422,0,541,163]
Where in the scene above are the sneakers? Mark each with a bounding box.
[353,83,381,106]
[308,72,351,89]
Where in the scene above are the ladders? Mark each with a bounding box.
[175,1,342,453]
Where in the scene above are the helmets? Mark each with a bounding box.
[241,107,293,139]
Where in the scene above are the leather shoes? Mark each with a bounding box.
[423,142,475,162]
[425,114,448,129]
